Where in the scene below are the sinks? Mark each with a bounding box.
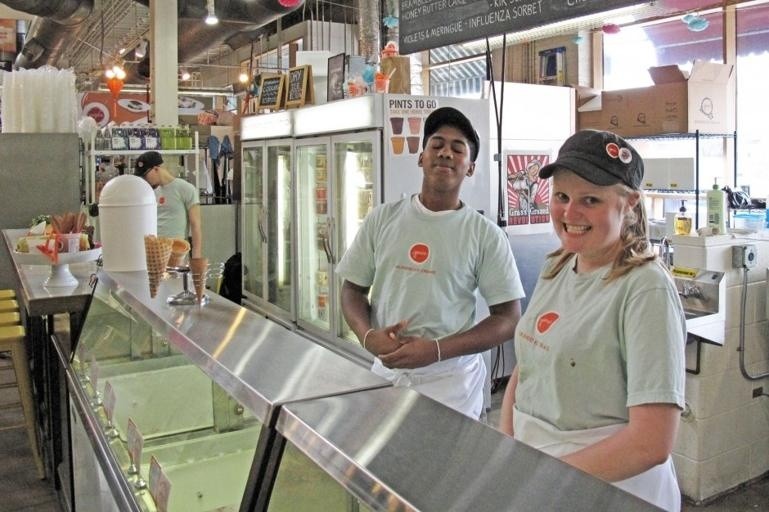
[683,308,713,323]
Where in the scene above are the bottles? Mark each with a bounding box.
[97,122,193,150]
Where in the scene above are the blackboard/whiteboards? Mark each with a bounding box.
[399,0,648,55]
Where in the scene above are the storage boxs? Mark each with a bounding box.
[577,59,734,138]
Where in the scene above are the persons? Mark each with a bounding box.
[500,127,687,511]
[133,150,204,260]
[332,106,527,420]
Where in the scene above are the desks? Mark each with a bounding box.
[3,229,94,490]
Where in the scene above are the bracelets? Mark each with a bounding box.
[434,337,443,362]
[362,327,375,349]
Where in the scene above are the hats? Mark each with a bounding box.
[133,151,163,176]
[538,128,645,190]
[423,106,480,161]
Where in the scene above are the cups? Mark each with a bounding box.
[342,79,390,98]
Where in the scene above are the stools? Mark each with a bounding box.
[1,287,49,482]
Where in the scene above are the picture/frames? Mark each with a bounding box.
[327,53,345,102]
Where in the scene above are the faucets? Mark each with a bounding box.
[682,282,692,299]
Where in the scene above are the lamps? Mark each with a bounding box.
[204,0,219,27]
[135,35,148,58]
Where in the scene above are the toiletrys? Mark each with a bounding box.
[675,201,692,234]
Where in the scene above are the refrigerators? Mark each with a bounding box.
[240,111,296,330]
[295,95,492,410]
